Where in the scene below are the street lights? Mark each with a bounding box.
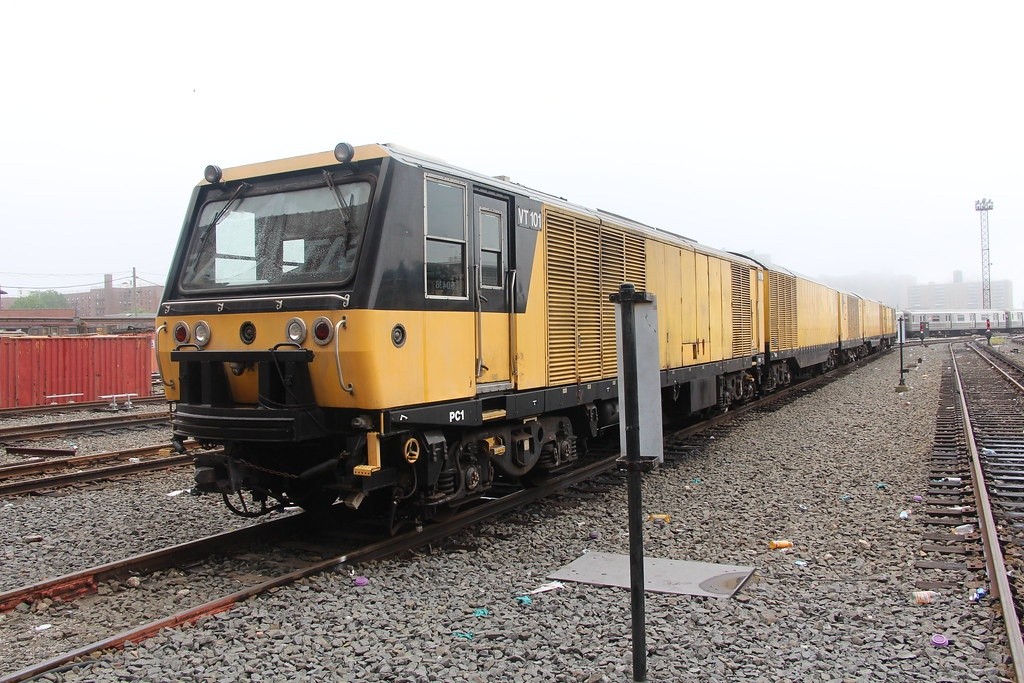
[974,199,994,308]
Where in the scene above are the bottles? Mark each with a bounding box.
[648,514,670,524]
[911,591,941,605]
[952,524,973,535]
[770,541,793,549]
[129,458,140,463]
[983,449,996,454]
[942,477,961,482]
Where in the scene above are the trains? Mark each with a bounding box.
[153,142,898,528]
[896,309,1024,336]
